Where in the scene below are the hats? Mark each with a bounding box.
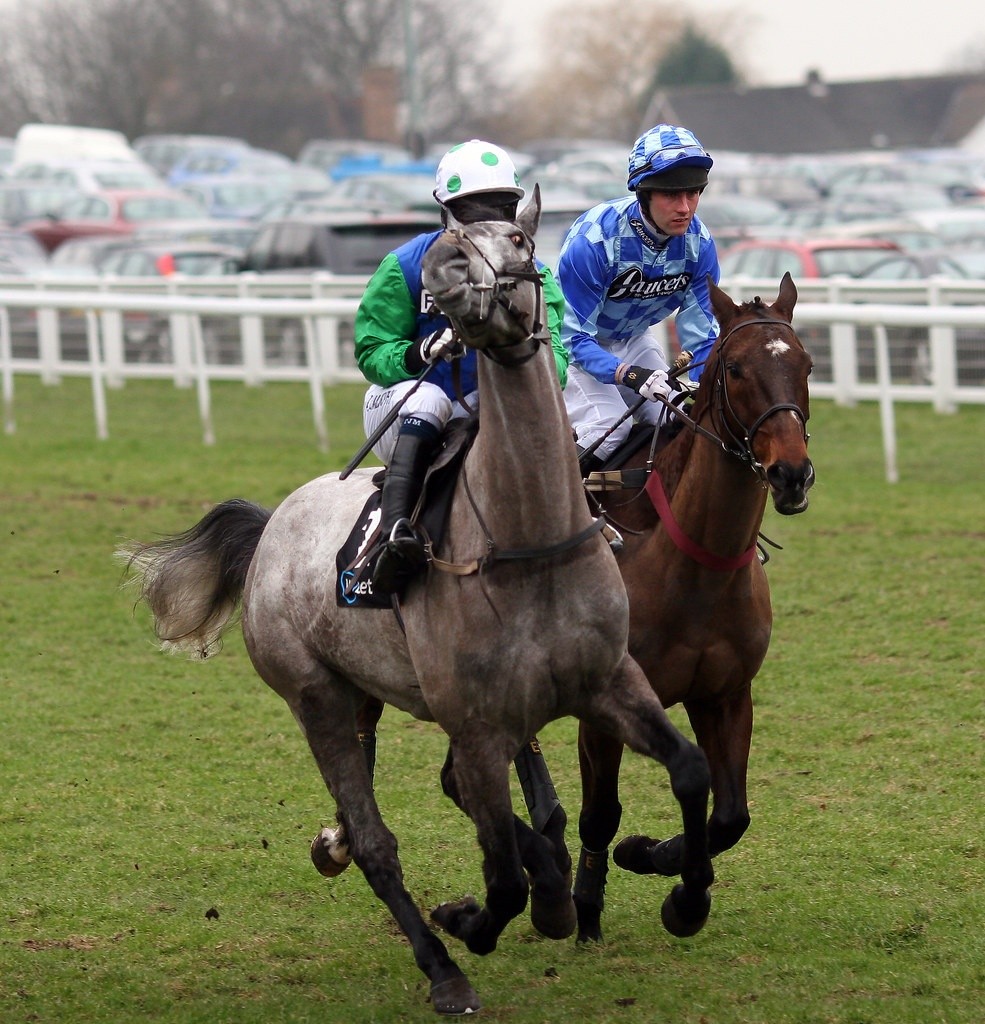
[436,139,525,205]
[635,162,709,196]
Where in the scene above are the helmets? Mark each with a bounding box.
[627,123,713,190]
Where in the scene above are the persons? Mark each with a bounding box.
[354,139,570,604]
[553,123,721,480]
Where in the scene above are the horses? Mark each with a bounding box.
[508,271,816,957]
[107,184,717,1022]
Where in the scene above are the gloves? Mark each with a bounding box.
[623,365,683,402]
[419,327,467,365]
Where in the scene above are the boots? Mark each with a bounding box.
[575,444,606,479]
[370,434,437,609]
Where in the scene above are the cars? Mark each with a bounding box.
[1,122,985,382]
